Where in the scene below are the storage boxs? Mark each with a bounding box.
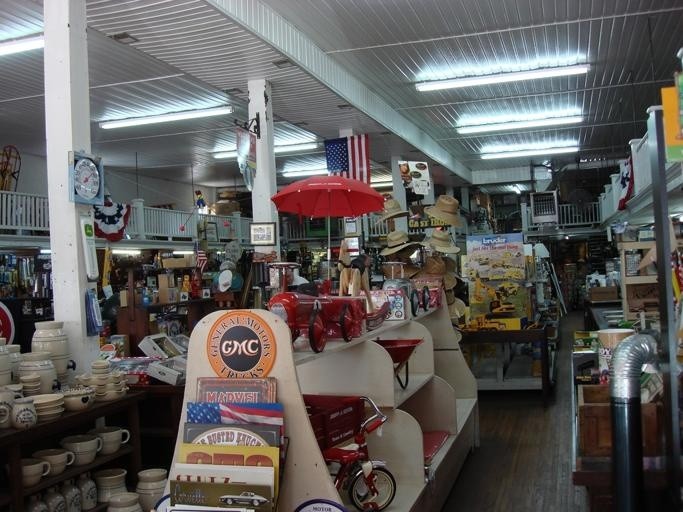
[578,381,662,457]
[589,287,619,301]
[299,392,365,451]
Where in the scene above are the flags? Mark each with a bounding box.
[323,133,370,185]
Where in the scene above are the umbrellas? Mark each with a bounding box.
[271,175,385,281]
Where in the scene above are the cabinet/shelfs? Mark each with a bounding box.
[454,260,564,404]
[0,383,151,512]
[176,274,481,511]
[112,292,216,360]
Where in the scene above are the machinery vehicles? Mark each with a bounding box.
[466,248,529,334]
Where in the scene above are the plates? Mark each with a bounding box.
[72,370,128,403]
[23,394,64,422]
[602,310,623,325]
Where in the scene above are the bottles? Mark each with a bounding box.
[28,467,167,512]
[0,319,70,395]
[0,384,37,429]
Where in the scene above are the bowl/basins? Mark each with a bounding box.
[64,389,95,409]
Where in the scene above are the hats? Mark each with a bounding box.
[375,200,410,225]
[411,256,457,291]
[424,195,462,228]
[441,257,464,282]
[451,319,466,333]
[420,231,460,254]
[379,230,420,259]
[445,289,465,319]
[382,255,420,278]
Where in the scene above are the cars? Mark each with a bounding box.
[216,491,267,507]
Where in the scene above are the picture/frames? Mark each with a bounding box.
[247,220,277,246]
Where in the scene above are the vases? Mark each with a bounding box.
[0,320,73,396]
[97,466,170,512]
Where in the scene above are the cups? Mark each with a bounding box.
[31,448,76,477]
[21,457,52,485]
[60,434,102,465]
[87,426,130,455]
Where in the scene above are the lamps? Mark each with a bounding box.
[99,103,237,131]
[410,61,591,93]
[477,146,581,160]
[455,114,588,136]
[208,140,392,192]
[1,30,46,58]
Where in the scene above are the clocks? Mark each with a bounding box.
[67,148,105,207]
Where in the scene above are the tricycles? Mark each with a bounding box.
[325,393,400,512]
